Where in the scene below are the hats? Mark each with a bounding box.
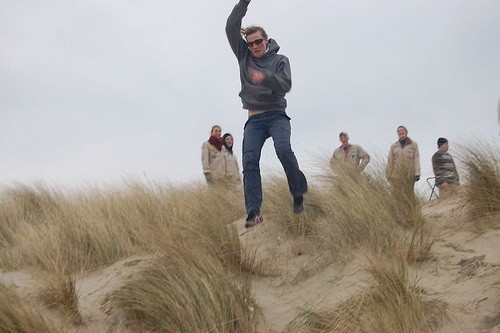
[438,138,448,147]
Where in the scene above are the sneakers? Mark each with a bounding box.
[244,211,263,227]
[294,196,304,213]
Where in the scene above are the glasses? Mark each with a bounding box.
[246,39,265,47]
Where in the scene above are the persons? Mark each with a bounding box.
[201,125,226,186]
[385,125,421,194]
[330,131,371,177]
[224,0,310,229]
[221,132,242,186]
[431,137,461,197]
